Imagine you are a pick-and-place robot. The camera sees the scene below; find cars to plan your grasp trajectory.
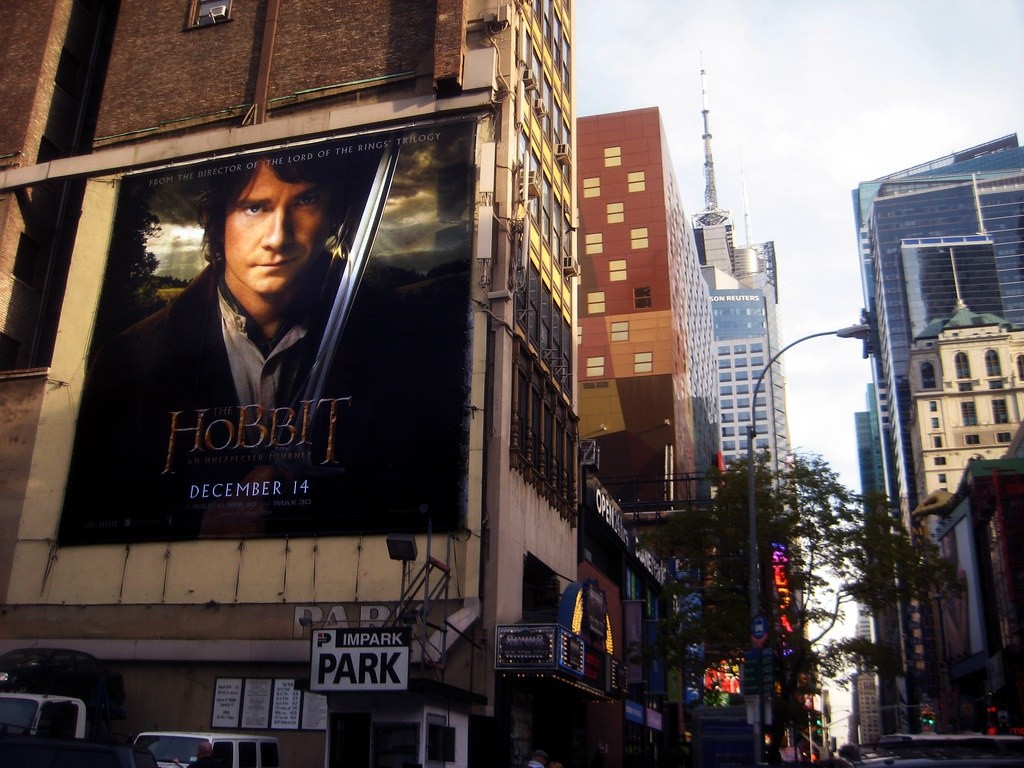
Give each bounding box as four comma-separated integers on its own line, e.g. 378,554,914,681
0,648,126,705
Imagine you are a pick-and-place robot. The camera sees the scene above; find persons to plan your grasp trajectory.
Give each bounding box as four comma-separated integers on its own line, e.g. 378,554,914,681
941,528,970,661
912,489,956,522
187,740,227,768
527,748,562,768
106,144,455,531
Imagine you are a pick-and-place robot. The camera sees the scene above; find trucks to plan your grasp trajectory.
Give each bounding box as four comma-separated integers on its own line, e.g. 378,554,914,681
0,692,87,740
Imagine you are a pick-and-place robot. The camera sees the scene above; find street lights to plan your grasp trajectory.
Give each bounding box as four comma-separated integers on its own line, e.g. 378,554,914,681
747,324,871,768
819,687,930,746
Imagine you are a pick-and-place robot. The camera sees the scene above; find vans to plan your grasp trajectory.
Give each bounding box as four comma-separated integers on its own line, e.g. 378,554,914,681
134,731,281,768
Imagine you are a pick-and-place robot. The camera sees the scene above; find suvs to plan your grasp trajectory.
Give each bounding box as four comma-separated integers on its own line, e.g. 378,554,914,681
0,723,157,768
